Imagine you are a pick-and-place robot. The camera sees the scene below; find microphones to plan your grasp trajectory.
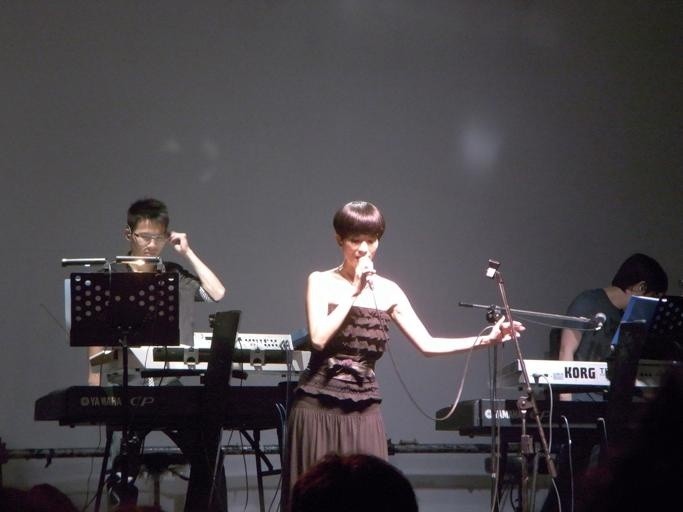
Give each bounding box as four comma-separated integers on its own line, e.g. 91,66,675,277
359,256,375,291
594,312,607,329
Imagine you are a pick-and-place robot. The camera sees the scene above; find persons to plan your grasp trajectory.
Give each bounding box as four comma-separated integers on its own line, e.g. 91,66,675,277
88,198,226,511
280,202,527,512
289,451,419,512
540,253,669,512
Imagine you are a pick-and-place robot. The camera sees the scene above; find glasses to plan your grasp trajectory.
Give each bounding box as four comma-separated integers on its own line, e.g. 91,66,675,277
133,231,166,241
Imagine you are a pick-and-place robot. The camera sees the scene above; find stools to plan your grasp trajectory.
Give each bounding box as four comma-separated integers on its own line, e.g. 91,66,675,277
114,452,190,511
486,453,557,511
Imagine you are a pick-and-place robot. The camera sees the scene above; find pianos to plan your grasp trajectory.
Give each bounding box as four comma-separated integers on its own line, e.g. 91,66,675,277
34,382,299,432
487,359,683,394
436,399,683,443
88,343,312,383
